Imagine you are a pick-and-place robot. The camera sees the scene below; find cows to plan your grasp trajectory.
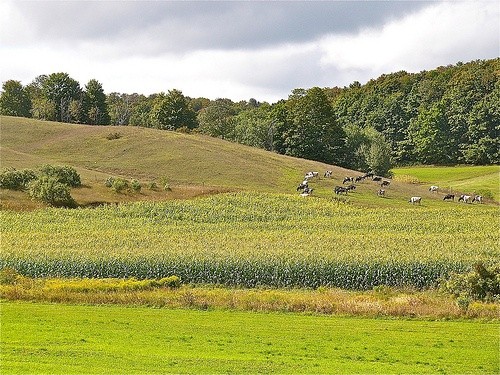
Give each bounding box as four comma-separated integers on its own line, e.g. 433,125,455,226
381,180,392,189
428,185,440,195
376,189,385,198
324,170,333,178
373,177,382,184
301,188,316,196
304,171,321,182
296,181,309,192
443,194,456,203
342,172,375,184
333,185,357,196
409,197,422,206
472,195,483,204
458,194,472,204
